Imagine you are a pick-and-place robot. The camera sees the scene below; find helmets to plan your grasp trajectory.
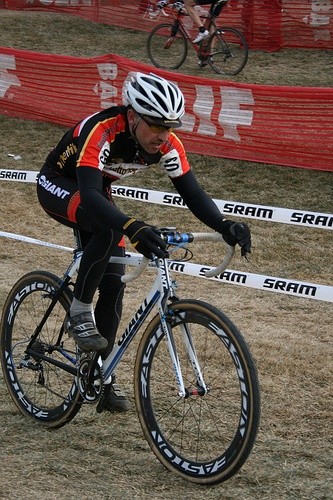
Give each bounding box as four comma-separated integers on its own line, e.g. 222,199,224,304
122,71,186,129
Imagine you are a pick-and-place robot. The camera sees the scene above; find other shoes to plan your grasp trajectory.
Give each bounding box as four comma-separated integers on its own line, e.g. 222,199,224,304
197,57,214,66
192,30,210,43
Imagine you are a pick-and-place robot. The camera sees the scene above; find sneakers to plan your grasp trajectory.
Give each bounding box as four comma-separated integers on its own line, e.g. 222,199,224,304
63,309,109,349
103,384,132,412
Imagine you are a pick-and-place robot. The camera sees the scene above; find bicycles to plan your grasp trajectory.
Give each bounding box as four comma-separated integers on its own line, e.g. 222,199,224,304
0,227,259,486
147,2,249,75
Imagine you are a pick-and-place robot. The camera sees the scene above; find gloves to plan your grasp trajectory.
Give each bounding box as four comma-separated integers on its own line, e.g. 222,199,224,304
216,220,251,257
117,217,167,259
156,1,166,10
173,2,182,10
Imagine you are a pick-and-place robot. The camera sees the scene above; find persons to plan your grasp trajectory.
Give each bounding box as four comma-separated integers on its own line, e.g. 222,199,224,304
37,73,251,409
157,0,226,65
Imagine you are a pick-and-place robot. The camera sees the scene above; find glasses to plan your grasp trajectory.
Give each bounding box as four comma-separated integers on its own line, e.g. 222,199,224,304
137,115,174,133
